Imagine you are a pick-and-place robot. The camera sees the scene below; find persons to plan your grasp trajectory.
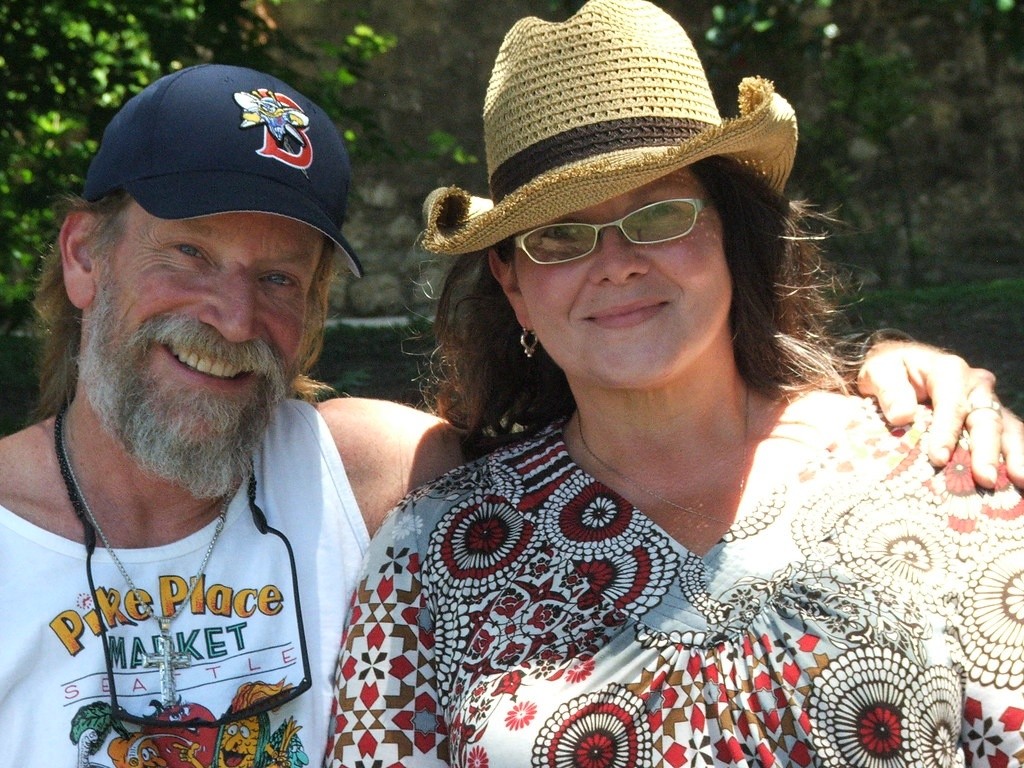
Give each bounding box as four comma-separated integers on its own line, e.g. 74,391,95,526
1,66,1023,768
323,0,1024,768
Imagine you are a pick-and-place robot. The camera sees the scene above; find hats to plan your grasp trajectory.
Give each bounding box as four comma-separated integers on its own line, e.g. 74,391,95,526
420,0,799,256
85,64,365,278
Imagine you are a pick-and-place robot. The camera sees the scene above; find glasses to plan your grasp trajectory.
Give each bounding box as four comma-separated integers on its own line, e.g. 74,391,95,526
78,502,312,728
514,196,717,265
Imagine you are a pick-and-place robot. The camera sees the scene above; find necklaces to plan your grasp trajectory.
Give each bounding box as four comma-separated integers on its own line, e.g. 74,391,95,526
577,385,749,525
60,420,235,709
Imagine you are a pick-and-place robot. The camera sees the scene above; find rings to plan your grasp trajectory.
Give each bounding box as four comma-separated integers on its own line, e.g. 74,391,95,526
967,399,1003,418
